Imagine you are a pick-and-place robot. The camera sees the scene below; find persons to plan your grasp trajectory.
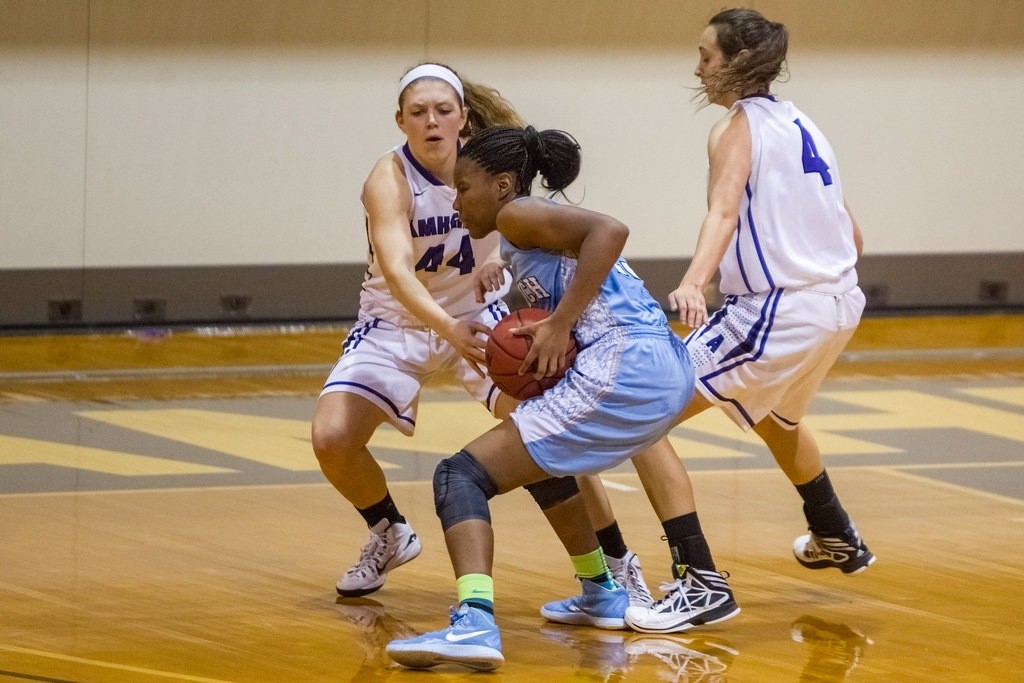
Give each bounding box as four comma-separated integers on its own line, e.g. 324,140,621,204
385,124,695,674
624,8,878,633
310,63,653,605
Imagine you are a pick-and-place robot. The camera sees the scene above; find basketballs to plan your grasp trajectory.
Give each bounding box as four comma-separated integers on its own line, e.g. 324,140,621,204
485,307,580,399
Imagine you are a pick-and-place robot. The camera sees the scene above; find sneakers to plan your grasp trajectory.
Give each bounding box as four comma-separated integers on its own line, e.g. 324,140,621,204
385,602,505,671
788,615,873,666
624,562,741,634
335,516,422,597
793,523,877,577
541,630,627,667
335,594,423,668
540,578,631,629
623,636,740,683
603,549,655,610
